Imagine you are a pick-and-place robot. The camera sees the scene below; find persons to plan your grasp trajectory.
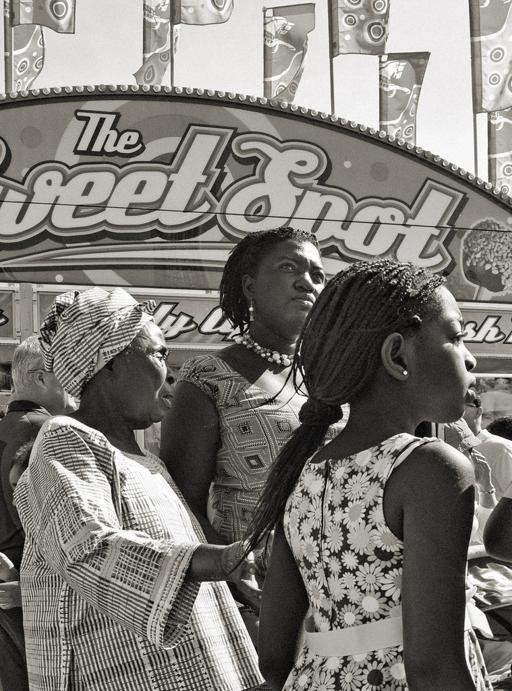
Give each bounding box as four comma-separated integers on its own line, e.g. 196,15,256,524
1,228,510,690
